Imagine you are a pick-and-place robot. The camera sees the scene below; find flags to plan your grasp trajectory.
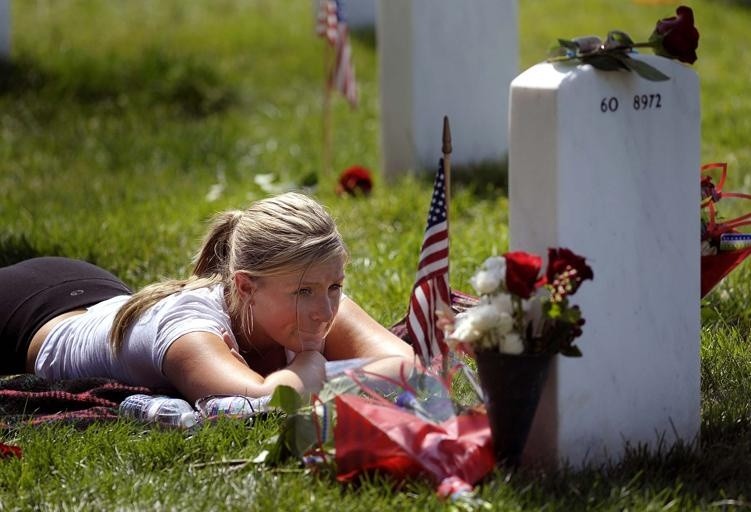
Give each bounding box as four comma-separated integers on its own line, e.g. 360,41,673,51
406,158,454,369
316,0,360,112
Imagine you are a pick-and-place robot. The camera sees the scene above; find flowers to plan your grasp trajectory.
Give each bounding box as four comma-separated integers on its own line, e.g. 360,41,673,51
335,165,373,199
443,245,594,481
254,345,495,511
701,161,751,302
542,7,700,82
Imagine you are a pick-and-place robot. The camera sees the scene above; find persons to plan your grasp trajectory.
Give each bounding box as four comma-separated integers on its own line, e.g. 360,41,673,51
0,190,423,412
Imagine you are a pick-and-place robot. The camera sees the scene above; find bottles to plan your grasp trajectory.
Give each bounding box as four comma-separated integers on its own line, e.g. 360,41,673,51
204,395,278,413
119,394,196,428
